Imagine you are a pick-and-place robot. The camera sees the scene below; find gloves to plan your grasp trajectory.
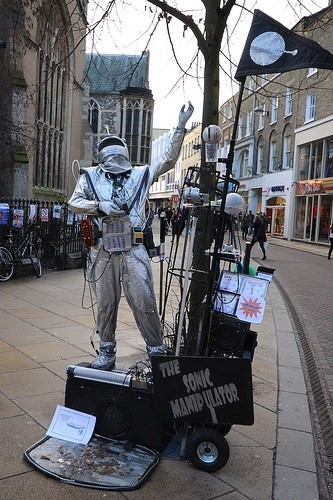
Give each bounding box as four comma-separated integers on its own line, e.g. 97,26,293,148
99,201,125,217
178,100,195,129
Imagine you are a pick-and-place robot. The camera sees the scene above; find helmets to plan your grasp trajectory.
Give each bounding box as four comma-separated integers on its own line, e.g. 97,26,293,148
97,136,132,173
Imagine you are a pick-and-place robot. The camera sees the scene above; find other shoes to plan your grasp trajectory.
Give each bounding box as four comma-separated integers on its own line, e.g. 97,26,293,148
92,340,117,370
262,256,267,260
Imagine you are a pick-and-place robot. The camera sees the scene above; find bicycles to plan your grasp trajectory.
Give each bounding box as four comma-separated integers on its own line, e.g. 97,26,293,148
0,226,42,282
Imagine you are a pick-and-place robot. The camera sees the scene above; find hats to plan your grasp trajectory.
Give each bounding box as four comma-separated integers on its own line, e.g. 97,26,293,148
255,215,261,221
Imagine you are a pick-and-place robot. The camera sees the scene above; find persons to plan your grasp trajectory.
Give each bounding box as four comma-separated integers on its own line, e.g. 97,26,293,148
148,206,269,243
328,221,333,260
67,101,194,371
251,215,267,260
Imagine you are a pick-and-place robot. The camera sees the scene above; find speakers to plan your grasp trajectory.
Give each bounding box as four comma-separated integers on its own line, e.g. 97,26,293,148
64,376,171,455
208,312,251,358
